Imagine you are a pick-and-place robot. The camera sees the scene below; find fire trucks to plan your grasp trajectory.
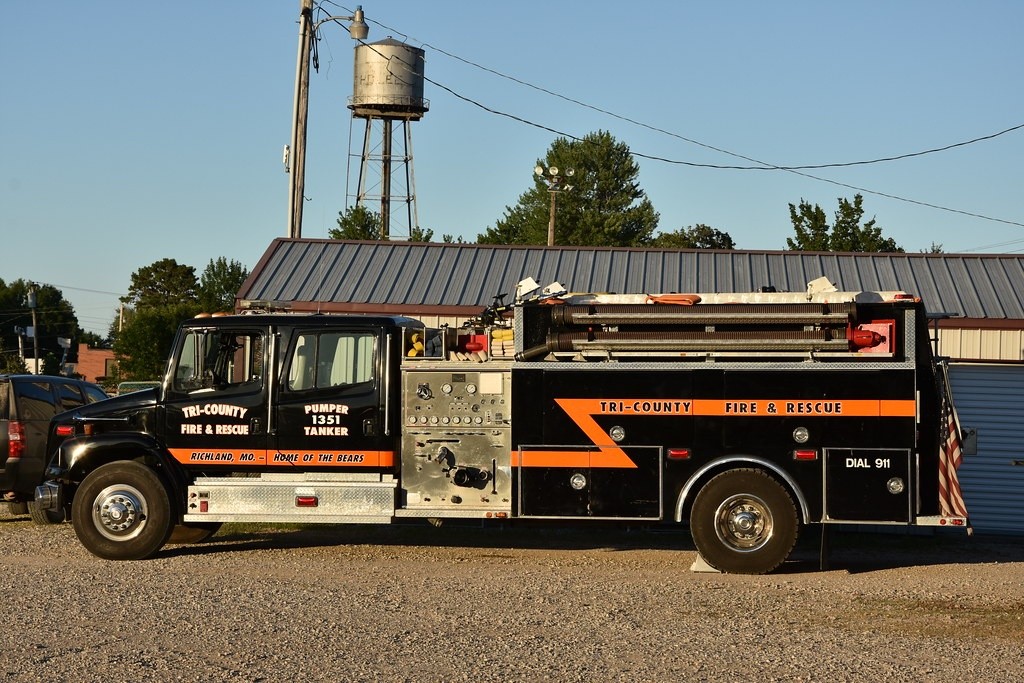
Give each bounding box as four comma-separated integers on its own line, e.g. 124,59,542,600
34,277,978,578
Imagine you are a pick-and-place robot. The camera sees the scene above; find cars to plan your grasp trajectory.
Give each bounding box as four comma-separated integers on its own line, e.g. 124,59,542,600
0,374,110,525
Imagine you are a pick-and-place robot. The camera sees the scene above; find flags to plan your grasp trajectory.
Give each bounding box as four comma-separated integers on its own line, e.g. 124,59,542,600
939,381,968,519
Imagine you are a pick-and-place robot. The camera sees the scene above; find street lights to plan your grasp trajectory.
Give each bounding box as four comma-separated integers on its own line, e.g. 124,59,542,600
290,4,369,239
533,165,576,247
